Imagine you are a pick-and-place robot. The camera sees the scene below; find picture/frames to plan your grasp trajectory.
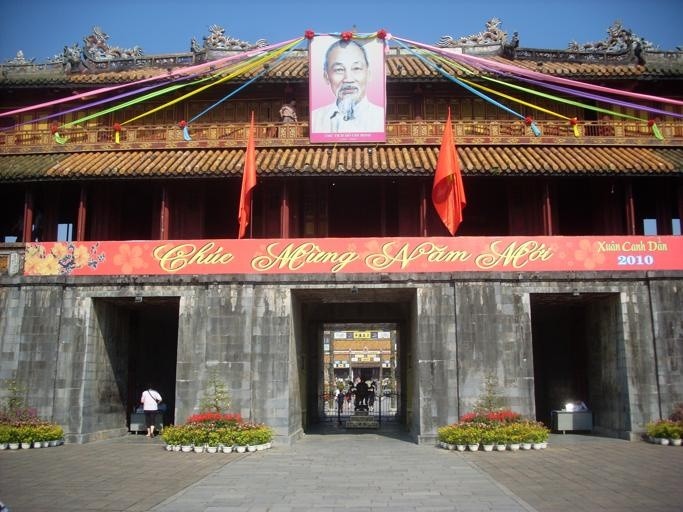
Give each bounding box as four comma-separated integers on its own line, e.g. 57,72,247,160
309,33,388,143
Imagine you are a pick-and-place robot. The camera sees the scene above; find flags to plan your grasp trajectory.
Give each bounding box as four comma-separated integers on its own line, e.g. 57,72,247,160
431,106,468,236
236,111,256,239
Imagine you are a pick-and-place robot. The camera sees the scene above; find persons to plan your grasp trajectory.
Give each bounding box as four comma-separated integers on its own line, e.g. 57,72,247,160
354,375,368,405
279,98,297,126
333,386,339,410
311,39,383,133
336,388,344,413
140,381,162,438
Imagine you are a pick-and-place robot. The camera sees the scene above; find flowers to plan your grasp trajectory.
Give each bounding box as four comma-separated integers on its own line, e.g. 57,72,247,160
439,372,551,445
159,366,271,447
0,379,64,443
641,402,683,439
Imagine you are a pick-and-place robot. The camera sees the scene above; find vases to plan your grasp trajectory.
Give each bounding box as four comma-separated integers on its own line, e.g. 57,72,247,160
647,435,683,446
167,442,273,453
441,443,548,450
0,441,64,450
